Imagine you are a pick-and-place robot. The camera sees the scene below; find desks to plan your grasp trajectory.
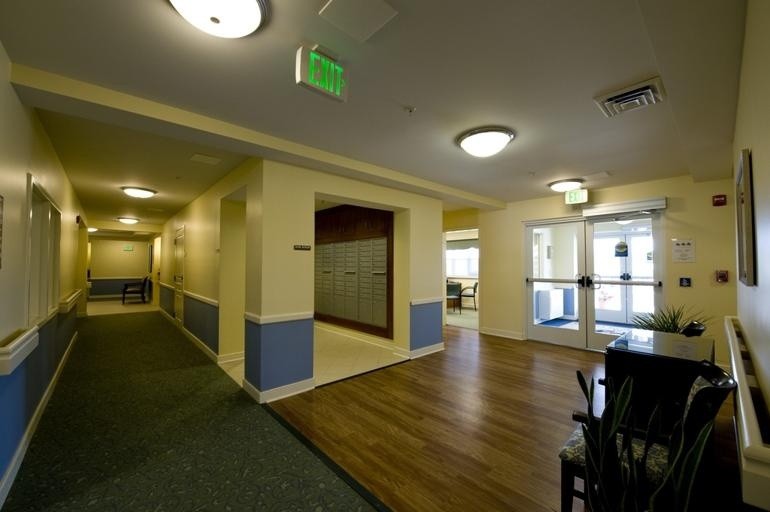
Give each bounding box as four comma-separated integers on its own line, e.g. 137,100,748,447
605,328,717,446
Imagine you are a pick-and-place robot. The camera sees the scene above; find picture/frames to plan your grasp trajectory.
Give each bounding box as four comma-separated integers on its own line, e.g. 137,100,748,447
734,146,755,289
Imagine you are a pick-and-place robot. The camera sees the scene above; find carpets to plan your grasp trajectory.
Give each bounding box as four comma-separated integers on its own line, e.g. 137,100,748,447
5,309,396,512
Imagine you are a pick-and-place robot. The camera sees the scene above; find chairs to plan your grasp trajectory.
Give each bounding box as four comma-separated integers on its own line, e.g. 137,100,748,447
121,274,150,306
556,357,738,512
444,282,479,315
679,318,707,337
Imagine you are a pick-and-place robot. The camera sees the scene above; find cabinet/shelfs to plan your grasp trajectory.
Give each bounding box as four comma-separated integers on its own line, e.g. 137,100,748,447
314,236,387,328
